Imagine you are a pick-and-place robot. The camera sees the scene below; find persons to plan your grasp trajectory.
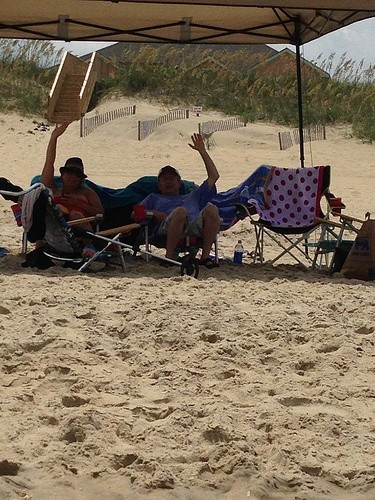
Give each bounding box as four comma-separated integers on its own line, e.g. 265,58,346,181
40,122,104,258
132,133,220,269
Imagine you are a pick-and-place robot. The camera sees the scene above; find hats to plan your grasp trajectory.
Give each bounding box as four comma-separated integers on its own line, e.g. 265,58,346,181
59,157,84,175
157,165,181,178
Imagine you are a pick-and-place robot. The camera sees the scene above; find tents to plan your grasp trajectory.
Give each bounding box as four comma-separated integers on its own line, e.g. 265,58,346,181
0,0,375,258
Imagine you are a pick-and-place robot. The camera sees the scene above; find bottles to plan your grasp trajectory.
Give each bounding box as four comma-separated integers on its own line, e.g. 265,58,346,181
11,204,22,226
97,251,107,263
233,240,244,263
365,212,370,221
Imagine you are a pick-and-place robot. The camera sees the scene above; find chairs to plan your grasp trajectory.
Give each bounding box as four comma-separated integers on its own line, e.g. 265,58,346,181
0,166,375,282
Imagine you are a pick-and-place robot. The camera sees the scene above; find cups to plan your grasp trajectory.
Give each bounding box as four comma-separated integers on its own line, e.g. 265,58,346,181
133,204,145,224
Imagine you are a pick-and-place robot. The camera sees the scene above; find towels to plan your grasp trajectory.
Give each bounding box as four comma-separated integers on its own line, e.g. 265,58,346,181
251,165,324,225
21,185,49,243
31,175,186,237
192,167,269,228
